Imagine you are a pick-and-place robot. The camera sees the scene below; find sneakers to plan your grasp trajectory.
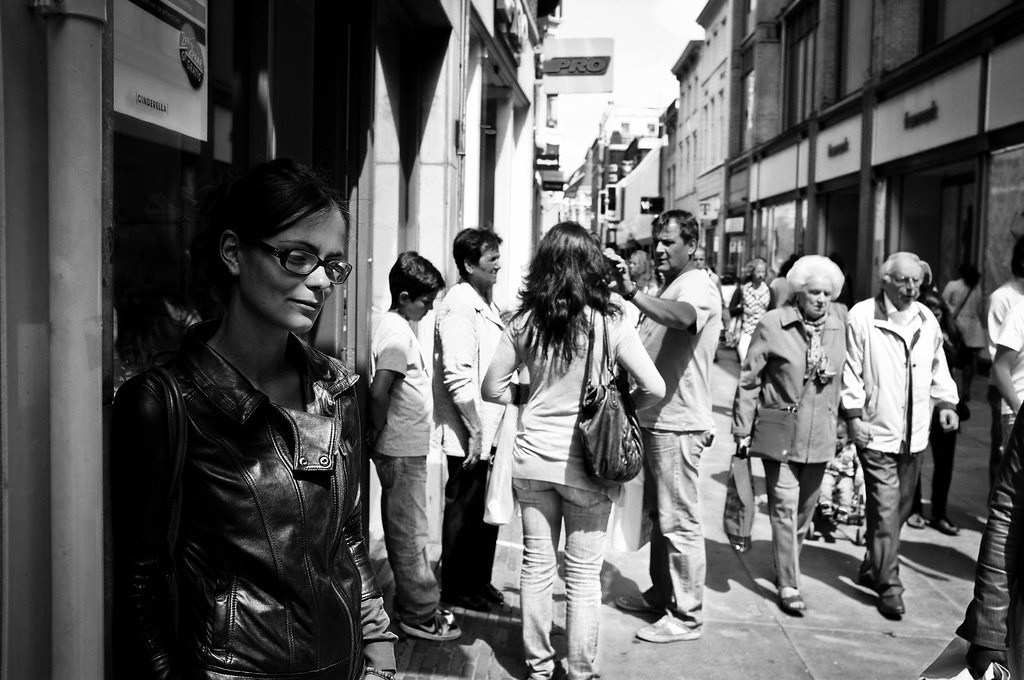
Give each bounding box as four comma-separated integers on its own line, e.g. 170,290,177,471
436,609,455,624
398,612,461,641
615,595,653,612
638,616,704,643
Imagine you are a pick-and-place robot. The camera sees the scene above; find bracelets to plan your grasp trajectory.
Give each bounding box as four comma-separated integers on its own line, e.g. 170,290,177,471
623,287,638,301
364,667,396,680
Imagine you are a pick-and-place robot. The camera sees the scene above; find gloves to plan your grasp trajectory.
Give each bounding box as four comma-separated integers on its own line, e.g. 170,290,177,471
965,643,1007,679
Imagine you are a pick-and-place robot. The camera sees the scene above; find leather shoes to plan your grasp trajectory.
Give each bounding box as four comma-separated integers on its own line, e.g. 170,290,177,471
858,577,884,595
879,595,905,613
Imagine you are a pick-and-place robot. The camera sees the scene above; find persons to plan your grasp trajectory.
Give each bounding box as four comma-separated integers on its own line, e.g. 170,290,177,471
840,252,960,614
954,401,1024,680
919,260,985,404
435,228,504,612
479,223,666,680
694,246,733,348
629,250,658,297
113,160,399,680
370,252,463,641
603,209,722,642
729,258,798,366
731,255,848,611
984,234,1024,482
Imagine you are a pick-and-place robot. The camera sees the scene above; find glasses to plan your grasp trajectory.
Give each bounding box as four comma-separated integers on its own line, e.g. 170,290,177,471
262,243,352,284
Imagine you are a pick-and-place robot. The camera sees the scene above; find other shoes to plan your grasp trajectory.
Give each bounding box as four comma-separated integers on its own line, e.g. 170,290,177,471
821,506,832,516
442,594,492,610
932,516,960,535
907,511,925,528
837,510,849,523
483,585,507,603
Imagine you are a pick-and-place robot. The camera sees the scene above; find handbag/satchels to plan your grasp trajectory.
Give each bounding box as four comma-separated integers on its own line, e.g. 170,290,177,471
104,366,198,680
750,407,798,463
577,306,644,481
619,467,652,550
728,287,744,316
723,446,754,552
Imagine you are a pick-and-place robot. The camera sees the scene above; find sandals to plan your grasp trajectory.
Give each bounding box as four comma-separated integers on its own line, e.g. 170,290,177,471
778,586,808,611
